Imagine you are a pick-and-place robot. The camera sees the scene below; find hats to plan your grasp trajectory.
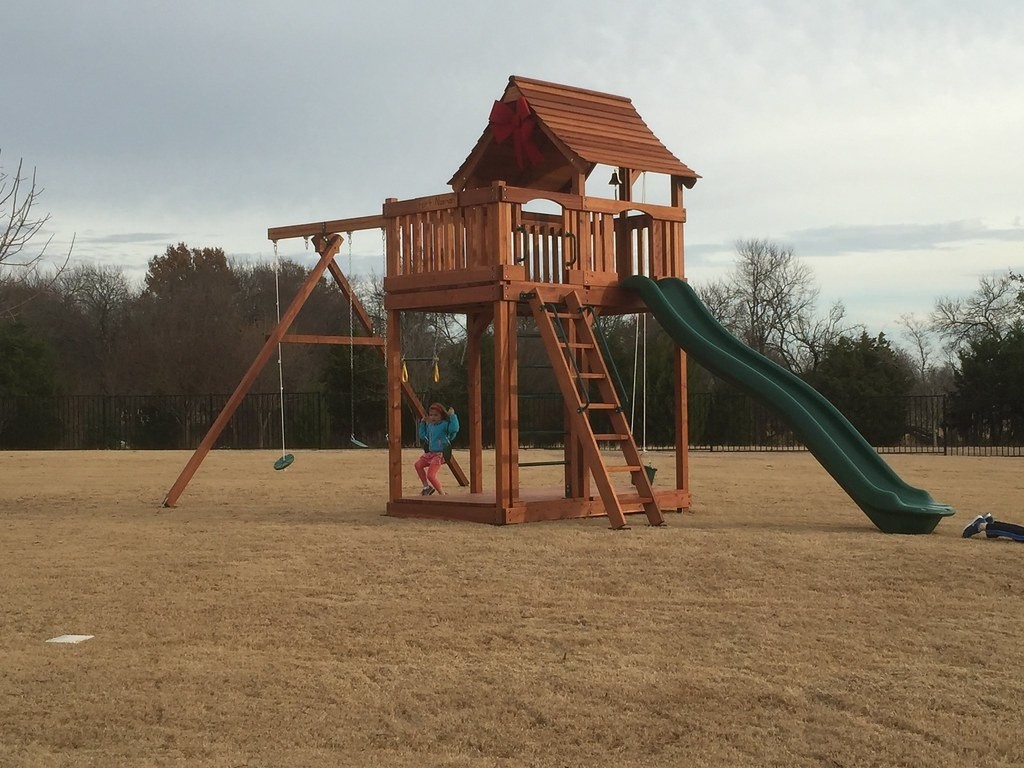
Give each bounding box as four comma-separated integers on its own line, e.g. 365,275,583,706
431,402,448,416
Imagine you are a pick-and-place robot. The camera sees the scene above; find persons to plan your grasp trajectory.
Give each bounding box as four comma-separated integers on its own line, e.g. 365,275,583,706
414,402,460,495
962,512,1024,543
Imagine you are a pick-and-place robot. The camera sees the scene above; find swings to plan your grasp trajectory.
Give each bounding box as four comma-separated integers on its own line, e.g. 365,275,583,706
347,226,389,448
424,312,470,465
273,235,310,470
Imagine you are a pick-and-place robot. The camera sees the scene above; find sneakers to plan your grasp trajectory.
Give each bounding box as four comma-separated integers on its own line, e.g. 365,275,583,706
962,515,986,538
421,485,435,495
984,513,995,523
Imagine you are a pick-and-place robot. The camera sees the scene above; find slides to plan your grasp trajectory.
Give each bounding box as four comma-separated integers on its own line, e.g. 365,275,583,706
626,271,960,537
